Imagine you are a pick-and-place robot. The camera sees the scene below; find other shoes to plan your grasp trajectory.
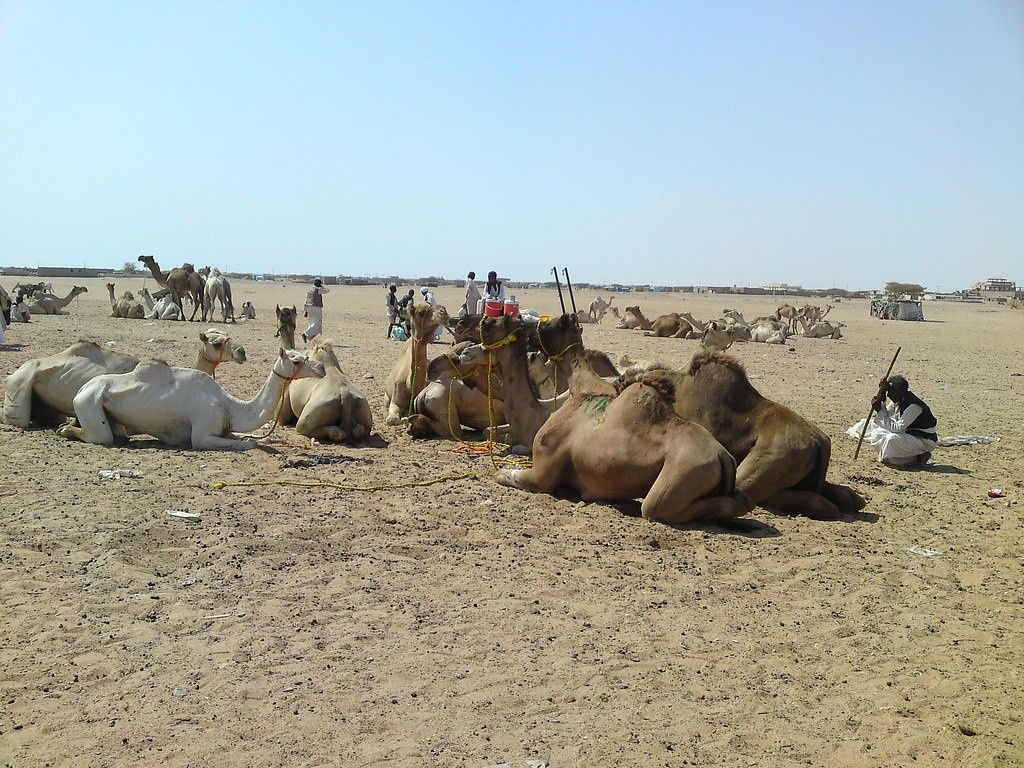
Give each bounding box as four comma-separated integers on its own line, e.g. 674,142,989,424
908,452,932,472
302,333,307,343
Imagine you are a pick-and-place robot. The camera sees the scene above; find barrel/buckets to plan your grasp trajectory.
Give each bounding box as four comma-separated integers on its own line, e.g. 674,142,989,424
485,299,502,318
504,300,519,320
540,315,549,322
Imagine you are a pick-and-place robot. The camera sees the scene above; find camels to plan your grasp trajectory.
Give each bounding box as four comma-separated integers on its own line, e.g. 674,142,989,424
578,297,845,354
272,304,374,443
56,348,328,451
383,302,867,521
0,328,247,429
106,255,256,324
12,279,89,315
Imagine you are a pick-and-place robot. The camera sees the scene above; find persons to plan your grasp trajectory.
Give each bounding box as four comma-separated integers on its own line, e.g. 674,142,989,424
386,285,414,339
872,375,937,465
420,287,442,339
10,298,31,322
240,301,256,319
302,279,329,342
483,271,505,307
220,301,235,318
382,282,387,288
458,272,481,316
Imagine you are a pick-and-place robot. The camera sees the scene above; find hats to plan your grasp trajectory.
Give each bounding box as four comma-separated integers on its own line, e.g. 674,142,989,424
887,375,909,395
420,286,428,293
